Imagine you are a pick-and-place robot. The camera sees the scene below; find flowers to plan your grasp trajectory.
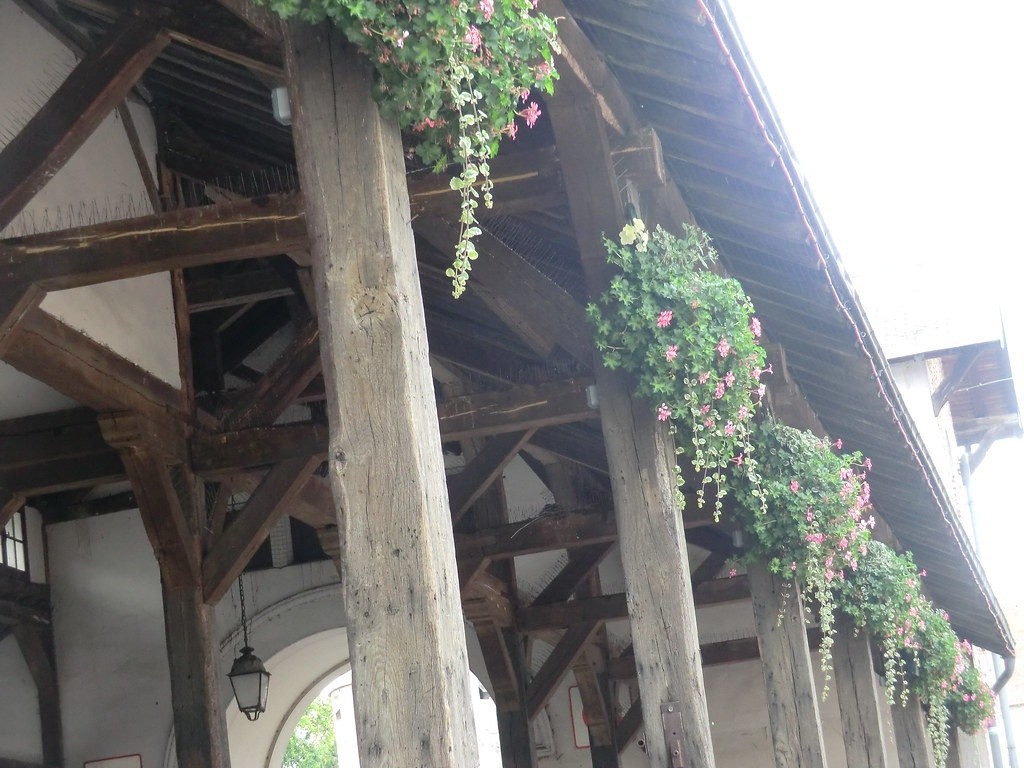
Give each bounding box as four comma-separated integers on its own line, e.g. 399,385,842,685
250,0,561,300
587,219,997,768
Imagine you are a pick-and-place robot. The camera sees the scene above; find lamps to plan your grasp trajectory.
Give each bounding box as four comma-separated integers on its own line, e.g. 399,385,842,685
226,477,271,721
